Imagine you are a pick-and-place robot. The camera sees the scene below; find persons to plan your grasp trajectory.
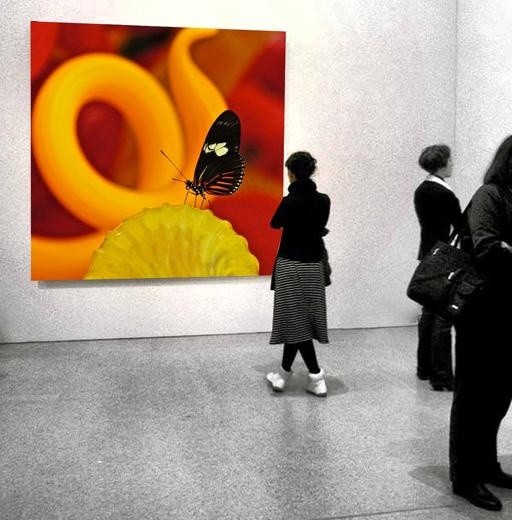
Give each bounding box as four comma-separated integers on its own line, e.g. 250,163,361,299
267,152,331,397
451,136,512,512
417,146,461,392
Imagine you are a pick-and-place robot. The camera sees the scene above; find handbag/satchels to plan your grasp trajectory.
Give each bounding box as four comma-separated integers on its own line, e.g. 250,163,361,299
405,239,493,324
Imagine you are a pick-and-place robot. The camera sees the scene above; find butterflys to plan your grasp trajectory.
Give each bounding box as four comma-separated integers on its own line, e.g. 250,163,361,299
160,109,248,210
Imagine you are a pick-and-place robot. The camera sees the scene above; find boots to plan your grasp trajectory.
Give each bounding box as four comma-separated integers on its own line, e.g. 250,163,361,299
305,368,327,398
267,366,294,393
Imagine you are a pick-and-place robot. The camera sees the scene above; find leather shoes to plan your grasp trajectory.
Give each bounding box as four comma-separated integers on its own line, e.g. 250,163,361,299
416,370,456,394
447,477,503,513
474,463,512,492
